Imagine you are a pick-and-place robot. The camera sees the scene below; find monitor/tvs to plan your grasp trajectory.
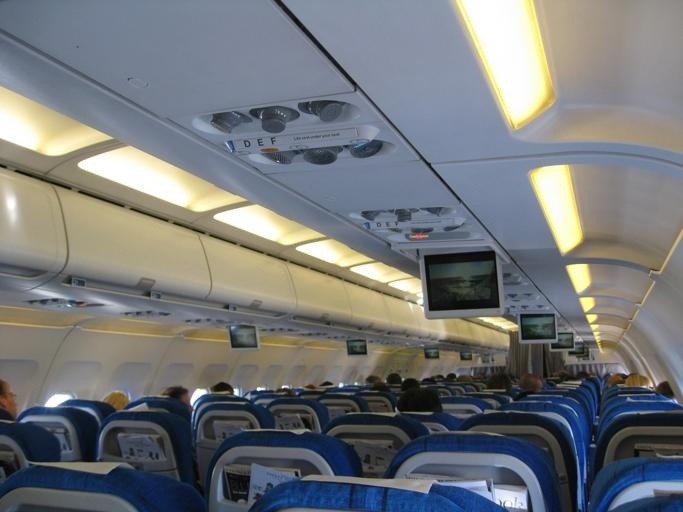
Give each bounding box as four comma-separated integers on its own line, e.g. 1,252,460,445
423,348,439,359
517,311,558,343
419,248,504,318
460,352,472,361
549,331,590,361
346,338,368,357
227,323,260,351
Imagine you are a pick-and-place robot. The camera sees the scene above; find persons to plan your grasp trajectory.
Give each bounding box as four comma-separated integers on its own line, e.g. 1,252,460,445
0,378,19,423
488,370,589,395
159,384,194,414
607,373,674,398
304,373,456,413
100,389,129,414
207,380,235,395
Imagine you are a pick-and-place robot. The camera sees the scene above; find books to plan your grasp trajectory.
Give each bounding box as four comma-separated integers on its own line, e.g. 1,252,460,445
273,414,308,431
224,437,532,512
48,424,69,451
0,449,20,481
631,443,683,456
115,431,168,460
211,418,252,441
